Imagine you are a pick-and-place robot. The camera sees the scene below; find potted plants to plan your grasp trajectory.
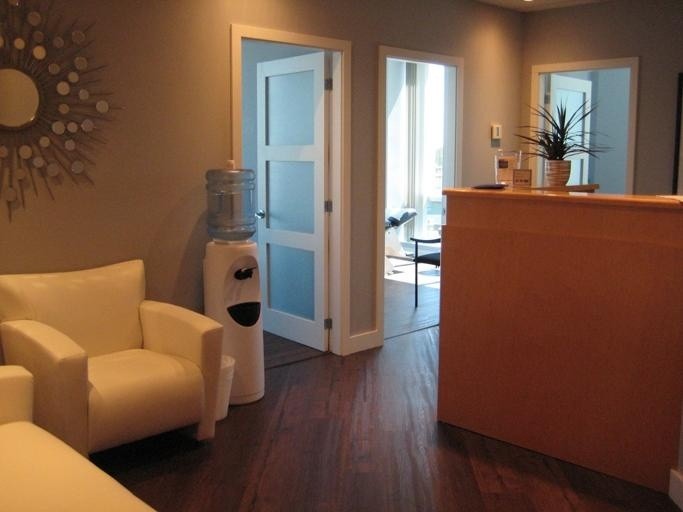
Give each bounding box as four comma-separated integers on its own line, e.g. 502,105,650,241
512,94,613,187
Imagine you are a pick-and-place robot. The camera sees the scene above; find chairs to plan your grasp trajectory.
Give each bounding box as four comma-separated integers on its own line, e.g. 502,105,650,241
1,257,224,461
1,361,155,511
408,234,442,307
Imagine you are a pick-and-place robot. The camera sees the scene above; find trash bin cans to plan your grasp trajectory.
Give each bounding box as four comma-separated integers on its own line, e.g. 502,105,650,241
216,355,235,422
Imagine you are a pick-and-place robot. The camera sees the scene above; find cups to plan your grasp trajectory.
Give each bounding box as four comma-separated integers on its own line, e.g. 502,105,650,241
494,149,521,187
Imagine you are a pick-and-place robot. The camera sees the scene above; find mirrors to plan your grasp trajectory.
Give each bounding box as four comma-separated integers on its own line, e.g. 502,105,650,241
0,63,45,133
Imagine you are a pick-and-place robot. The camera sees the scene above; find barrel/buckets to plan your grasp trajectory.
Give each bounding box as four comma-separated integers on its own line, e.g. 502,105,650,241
205,168,256,241
